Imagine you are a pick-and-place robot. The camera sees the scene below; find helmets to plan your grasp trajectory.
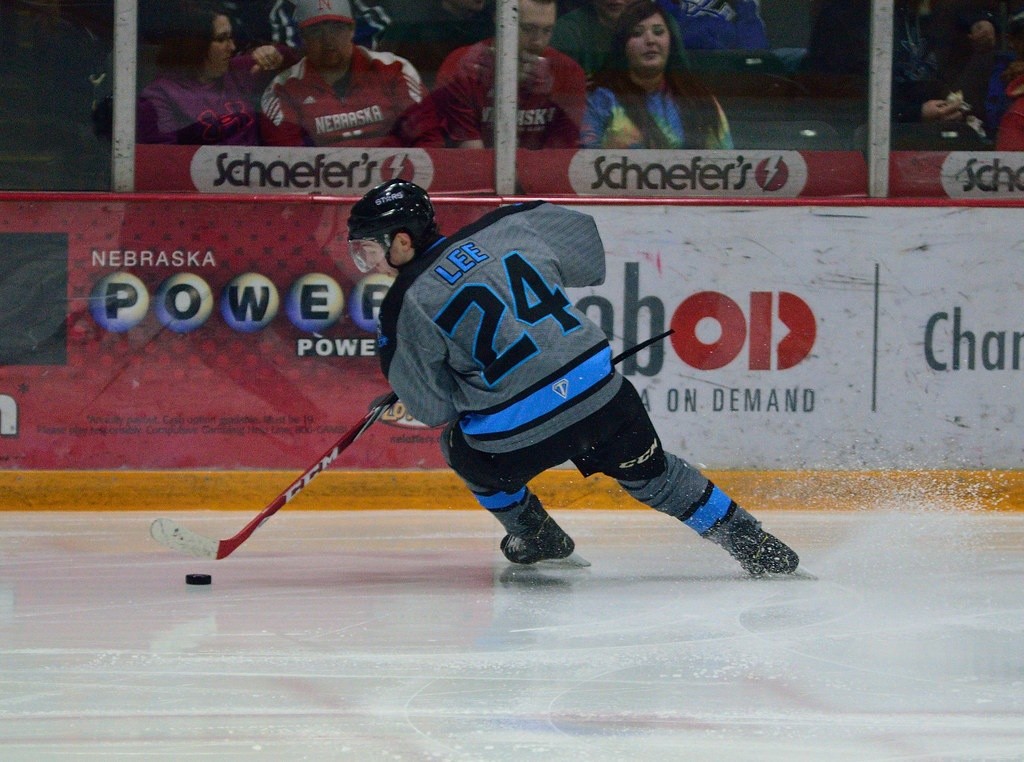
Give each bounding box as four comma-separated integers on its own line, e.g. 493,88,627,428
348,179,438,248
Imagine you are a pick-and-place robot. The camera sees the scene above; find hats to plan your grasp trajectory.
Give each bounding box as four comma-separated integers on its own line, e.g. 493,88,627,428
291,0,355,29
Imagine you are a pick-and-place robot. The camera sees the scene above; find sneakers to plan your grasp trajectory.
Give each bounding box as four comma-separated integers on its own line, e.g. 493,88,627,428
740,535,799,574
498,516,575,565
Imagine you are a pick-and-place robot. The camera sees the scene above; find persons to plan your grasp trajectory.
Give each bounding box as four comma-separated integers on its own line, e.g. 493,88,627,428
136,1,300,146
576,0,735,150
60,0,1024,149
257,0,445,149
433,0,588,149
345,176,815,583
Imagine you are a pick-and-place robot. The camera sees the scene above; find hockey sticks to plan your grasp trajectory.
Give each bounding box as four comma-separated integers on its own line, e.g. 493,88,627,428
148,390,400,562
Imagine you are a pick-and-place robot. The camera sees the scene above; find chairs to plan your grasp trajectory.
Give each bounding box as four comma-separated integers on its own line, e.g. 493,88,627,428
689,47,783,76
851,122,979,151
729,118,842,153
0,12,95,157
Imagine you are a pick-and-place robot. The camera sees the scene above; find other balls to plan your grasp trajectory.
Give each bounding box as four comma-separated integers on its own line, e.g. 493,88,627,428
185,573,212,586
